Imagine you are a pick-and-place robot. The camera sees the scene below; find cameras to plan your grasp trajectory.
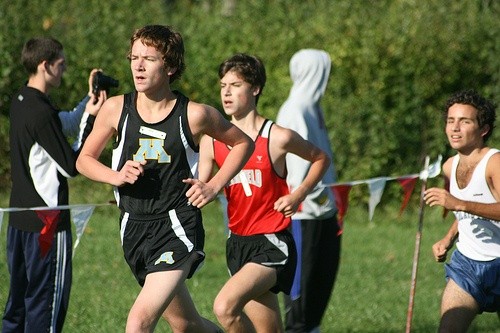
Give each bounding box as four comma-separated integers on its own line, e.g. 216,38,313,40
92,72,119,105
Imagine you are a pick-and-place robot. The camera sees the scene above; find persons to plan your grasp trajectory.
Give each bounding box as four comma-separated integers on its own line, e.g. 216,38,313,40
197,53,330,333
277,49,342,333
3,36,108,333
75,26,254,333
421,90,500,333
